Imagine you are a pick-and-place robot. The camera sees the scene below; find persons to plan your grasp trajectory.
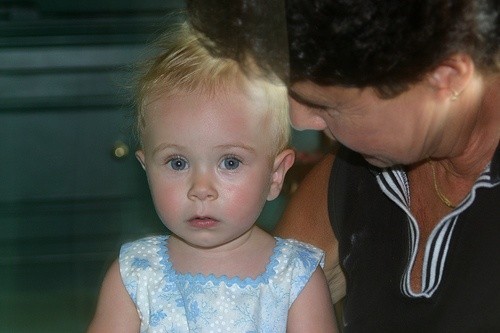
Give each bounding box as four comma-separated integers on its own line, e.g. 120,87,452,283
86,20,340,333
180,0,500,333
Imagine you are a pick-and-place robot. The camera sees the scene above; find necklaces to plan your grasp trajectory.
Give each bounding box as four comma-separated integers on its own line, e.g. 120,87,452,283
426,155,469,209
